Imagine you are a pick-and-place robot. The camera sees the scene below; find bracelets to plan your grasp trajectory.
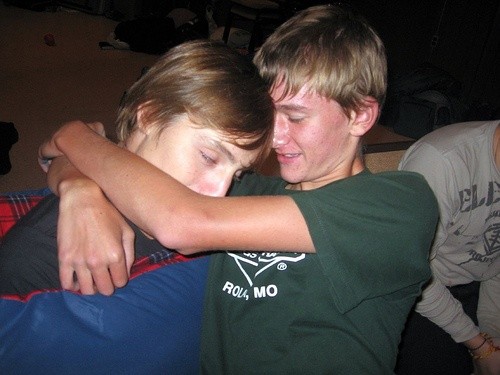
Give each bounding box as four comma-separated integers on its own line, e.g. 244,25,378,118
467,333,495,361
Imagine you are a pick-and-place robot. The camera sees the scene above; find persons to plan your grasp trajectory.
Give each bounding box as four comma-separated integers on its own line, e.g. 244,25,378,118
395,118,500,375
37,4,438,375
0,36,275,298
